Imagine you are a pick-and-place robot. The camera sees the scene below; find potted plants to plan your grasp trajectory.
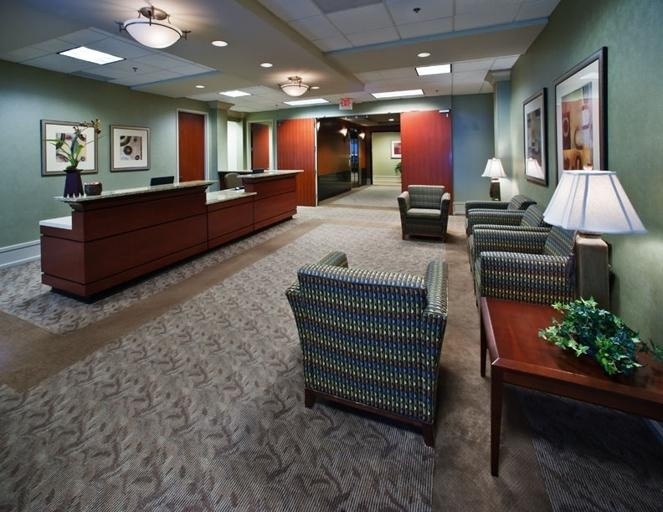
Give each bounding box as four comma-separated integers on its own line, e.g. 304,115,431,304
44,117,103,171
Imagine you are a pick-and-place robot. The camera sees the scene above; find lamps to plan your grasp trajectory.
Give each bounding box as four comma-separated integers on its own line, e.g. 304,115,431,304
541,163,649,314
478,156,506,201
112,7,189,49
276,75,311,98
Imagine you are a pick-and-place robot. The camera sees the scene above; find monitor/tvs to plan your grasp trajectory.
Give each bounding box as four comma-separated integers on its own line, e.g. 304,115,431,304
253,169,264,174
151,176,174,186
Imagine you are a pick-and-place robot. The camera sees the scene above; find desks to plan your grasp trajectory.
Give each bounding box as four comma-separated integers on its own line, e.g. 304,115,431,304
474,292,662,479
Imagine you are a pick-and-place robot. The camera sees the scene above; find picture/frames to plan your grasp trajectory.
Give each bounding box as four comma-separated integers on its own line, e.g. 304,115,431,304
551,45,610,191
37,118,99,180
104,122,151,173
389,138,402,160
520,85,550,191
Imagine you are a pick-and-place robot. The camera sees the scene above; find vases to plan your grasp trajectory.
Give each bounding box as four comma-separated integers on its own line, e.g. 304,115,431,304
62,168,85,198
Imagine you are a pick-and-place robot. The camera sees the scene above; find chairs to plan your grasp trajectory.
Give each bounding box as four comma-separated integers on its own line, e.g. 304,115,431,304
284,250,450,453
467,223,579,306
463,191,538,238
394,184,453,243
224,171,243,189
466,203,548,271
